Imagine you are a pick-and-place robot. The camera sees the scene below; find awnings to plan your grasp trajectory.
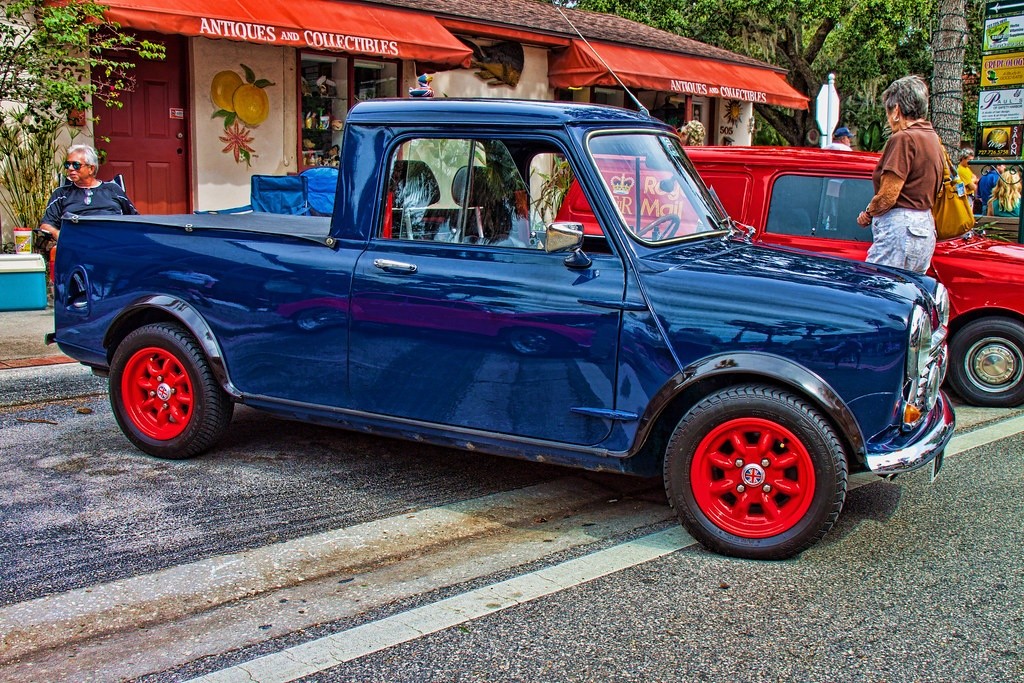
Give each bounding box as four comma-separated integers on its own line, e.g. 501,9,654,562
35,0,473,69
548,39,810,112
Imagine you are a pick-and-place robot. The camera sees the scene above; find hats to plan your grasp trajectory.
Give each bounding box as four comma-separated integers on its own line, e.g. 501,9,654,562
835,127,854,138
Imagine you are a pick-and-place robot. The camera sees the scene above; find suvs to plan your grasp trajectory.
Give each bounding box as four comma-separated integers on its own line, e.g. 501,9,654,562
549,144,1024,409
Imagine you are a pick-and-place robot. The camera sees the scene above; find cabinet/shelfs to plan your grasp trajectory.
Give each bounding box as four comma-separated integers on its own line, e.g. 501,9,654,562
296,47,404,173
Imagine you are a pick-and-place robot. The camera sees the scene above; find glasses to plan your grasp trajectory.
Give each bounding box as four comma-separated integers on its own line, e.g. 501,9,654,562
63,161,90,170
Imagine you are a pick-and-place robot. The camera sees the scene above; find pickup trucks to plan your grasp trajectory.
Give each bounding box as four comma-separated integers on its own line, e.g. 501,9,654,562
44,7,958,562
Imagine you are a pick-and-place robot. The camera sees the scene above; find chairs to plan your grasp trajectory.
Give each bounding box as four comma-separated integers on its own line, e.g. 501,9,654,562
194,175,311,216
33,173,125,260
389,160,439,240
451,166,503,237
782,208,813,235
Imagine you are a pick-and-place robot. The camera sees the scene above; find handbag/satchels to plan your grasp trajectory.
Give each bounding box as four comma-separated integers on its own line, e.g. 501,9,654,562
932,137,975,239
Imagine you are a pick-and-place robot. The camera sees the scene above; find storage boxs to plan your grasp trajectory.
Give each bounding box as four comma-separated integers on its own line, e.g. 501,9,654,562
0,253,47,311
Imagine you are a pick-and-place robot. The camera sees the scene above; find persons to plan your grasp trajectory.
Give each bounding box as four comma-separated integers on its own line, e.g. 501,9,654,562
956,148,980,215
856,75,946,275
977,156,1007,216
987,171,1022,217
821,128,856,151
40,144,141,244
